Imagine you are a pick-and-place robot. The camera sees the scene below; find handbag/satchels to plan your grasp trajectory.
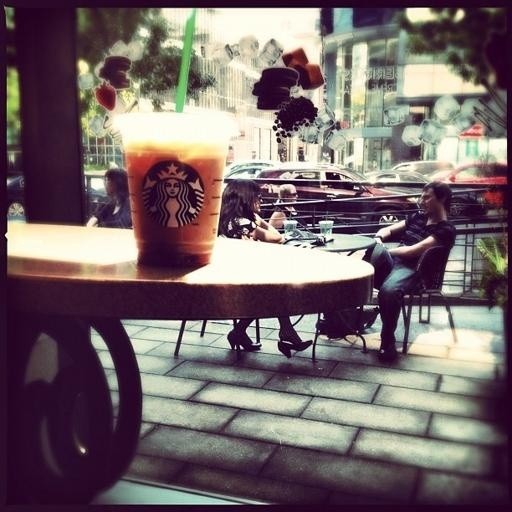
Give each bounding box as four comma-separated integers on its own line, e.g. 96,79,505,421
322,307,380,339
376,340,396,362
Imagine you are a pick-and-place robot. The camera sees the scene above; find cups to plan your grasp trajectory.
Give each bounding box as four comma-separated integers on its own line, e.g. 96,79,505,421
282,220,298,236
319,220,334,238
113,108,237,266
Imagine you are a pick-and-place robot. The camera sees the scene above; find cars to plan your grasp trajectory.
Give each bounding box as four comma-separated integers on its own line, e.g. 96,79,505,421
224,160,507,232
8,175,110,218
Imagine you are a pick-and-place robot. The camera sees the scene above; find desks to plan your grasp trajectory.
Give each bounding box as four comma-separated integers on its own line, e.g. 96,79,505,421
279,228,375,363
0,223,374,511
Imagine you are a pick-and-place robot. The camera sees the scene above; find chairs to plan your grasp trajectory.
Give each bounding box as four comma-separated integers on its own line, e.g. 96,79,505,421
374,234,454,355
175,218,260,357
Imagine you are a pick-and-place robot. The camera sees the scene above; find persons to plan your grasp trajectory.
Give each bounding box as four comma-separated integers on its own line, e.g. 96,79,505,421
6,156,25,215
217,179,313,358
363,181,456,362
268,183,308,234
86,168,132,227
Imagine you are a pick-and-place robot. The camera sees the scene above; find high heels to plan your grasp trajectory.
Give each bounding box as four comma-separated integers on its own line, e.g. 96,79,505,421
277,340,312,358
227,330,262,352
